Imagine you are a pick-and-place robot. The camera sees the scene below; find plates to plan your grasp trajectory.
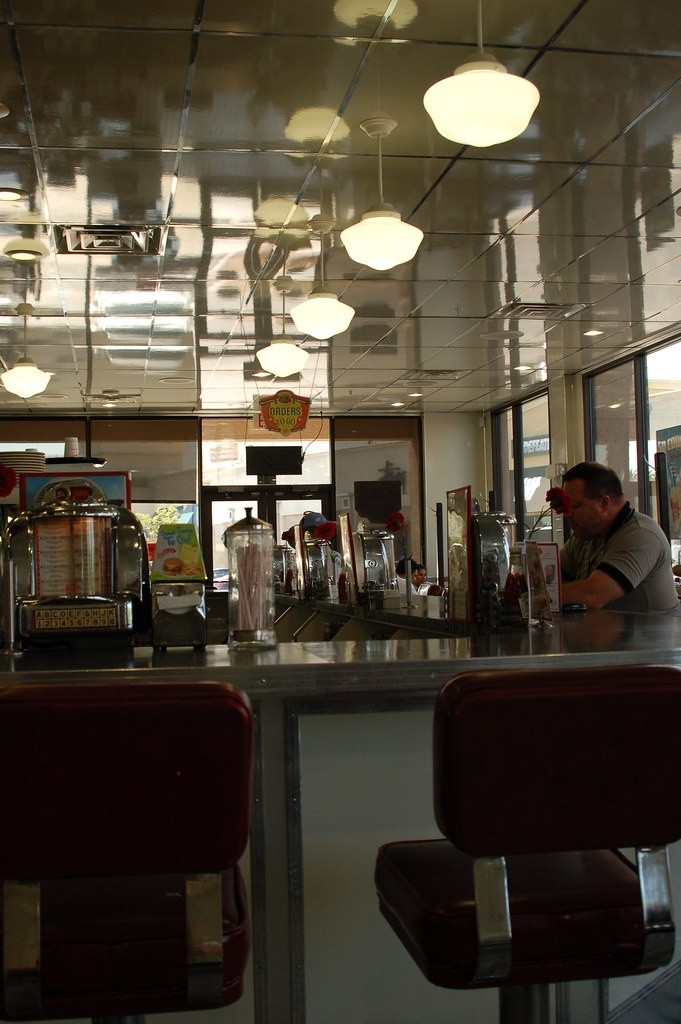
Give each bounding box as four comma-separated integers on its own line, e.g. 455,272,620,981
0,451,47,483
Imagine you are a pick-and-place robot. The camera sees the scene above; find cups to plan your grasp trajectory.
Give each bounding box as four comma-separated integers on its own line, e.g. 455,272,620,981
64,437,80,457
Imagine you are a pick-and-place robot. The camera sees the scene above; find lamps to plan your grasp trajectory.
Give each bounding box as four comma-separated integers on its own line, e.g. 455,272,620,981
422,0,540,149
340,119,424,270
290,221,356,340
256,282,310,377
0,307,51,399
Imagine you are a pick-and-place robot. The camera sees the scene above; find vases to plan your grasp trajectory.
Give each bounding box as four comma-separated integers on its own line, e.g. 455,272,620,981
333,561,336,581
401,559,419,609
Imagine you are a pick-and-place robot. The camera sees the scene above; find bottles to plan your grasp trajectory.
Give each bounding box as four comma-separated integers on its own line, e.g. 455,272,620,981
338,566,349,603
225,508,278,650
285,561,296,596
503,547,529,629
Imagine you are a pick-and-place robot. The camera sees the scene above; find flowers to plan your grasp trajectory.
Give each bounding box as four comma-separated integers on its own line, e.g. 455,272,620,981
385,511,413,558
281,531,292,551
523,487,573,540
314,521,338,562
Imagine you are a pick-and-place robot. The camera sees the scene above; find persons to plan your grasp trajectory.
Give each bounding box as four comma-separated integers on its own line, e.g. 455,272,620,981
389,558,418,595
413,564,431,596
428,585,447,597
309,548,353,582
559,462,681,616
550,610,676,653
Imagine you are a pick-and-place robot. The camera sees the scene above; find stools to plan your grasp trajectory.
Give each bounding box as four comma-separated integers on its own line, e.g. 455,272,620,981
375,664,681,1024
0,680,255,1024
